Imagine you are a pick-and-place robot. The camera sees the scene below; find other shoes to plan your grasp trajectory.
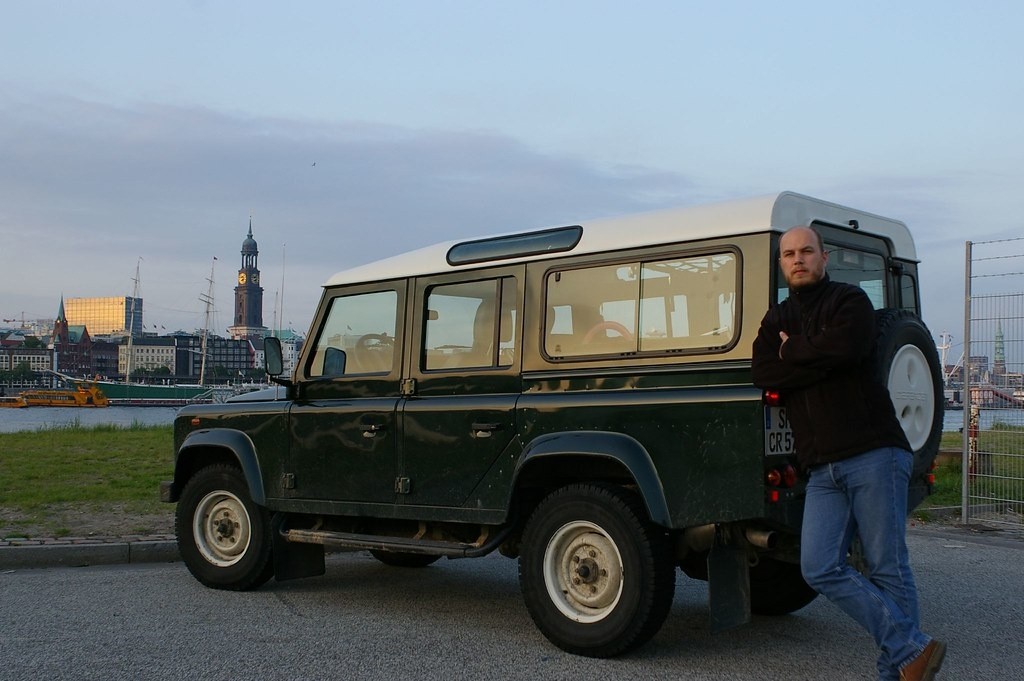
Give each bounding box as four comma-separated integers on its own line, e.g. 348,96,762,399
899,639,947,681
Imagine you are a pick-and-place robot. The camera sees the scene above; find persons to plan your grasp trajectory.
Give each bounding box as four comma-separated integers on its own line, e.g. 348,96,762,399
749,227,948,681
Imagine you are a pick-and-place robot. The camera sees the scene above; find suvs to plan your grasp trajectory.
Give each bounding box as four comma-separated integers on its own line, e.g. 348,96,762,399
157,189,945,659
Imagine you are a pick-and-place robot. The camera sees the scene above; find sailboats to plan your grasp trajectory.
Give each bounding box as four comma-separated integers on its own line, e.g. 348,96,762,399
0,251,261,409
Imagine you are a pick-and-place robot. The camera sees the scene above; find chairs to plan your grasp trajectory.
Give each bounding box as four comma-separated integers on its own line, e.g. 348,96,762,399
442,297,607,368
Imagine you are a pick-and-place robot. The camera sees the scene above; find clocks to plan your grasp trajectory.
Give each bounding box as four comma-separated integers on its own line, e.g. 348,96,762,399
238,273,247,284
251,273,258,284
